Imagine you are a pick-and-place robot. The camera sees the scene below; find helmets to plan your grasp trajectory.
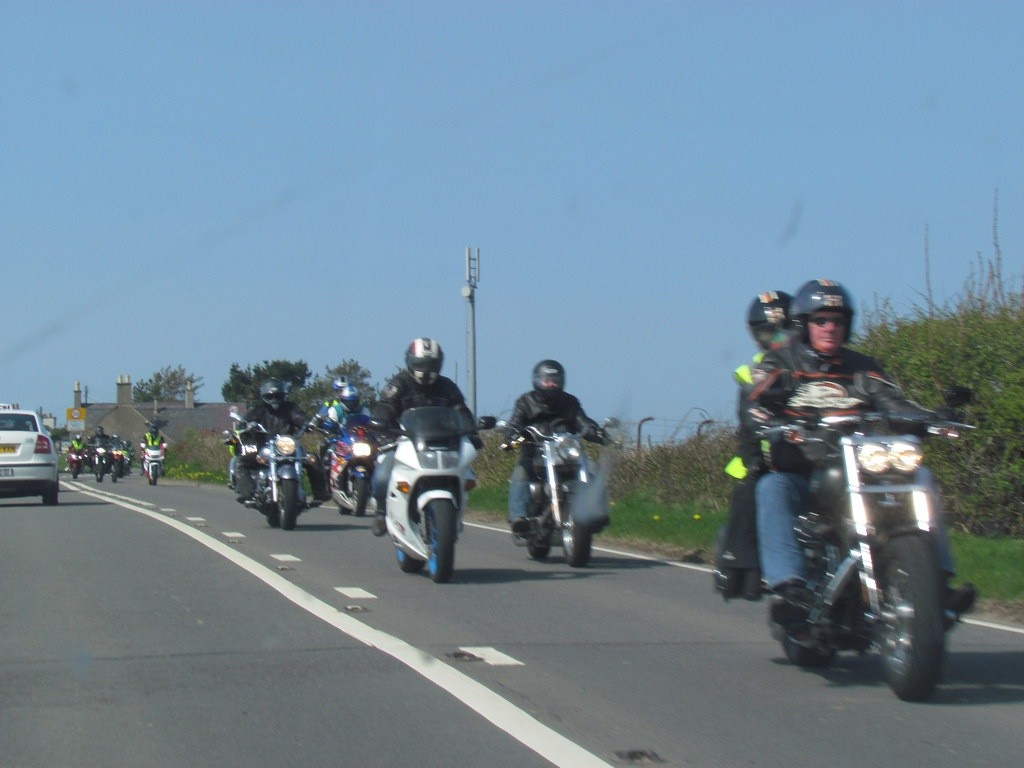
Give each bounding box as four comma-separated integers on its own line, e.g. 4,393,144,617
405,337,443,385
748,290,798,351
112,433,119,439
334,377,350,391
532,360,564,399
341,386,360,407
95,426,103,435
260,377,284,406
76,434,81,441
150,425,158,433
792,279,855,322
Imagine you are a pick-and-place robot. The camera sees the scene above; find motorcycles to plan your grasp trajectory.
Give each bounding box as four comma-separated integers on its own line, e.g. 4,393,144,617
140,442,168,485
489,414,624,568
223,404,328,530
66,437,136,484
748,385,986,704
312,403,380,518
356,401,499,583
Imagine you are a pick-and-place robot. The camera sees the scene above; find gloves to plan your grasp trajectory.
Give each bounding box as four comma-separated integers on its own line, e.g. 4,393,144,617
934,407,957,424
765,419,810,443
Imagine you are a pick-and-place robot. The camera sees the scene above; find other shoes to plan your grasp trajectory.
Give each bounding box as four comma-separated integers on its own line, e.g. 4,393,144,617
741,570,761,600
371,510,387,538
770,596,815,634
314,493,333,501
938,582,977,632
236,497,253,503
512,515,531,539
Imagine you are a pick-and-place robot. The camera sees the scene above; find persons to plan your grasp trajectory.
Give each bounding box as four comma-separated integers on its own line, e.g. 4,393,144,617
711,279,979,632
139,424,167,476
110,434,134,475
228,421,240,487
66,435,89,472
88,427,114,474
369,336,483,537
305,375,375,501
504,360,611,536
233,377,312,504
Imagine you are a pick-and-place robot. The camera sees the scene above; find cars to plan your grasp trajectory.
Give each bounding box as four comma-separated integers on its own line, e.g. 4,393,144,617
0,404,60,507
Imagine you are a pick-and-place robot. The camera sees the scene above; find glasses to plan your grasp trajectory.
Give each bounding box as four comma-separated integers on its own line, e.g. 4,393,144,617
804,314,847,327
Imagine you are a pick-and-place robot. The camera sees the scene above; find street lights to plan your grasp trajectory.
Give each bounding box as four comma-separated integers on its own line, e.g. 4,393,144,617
460,245,482,417
